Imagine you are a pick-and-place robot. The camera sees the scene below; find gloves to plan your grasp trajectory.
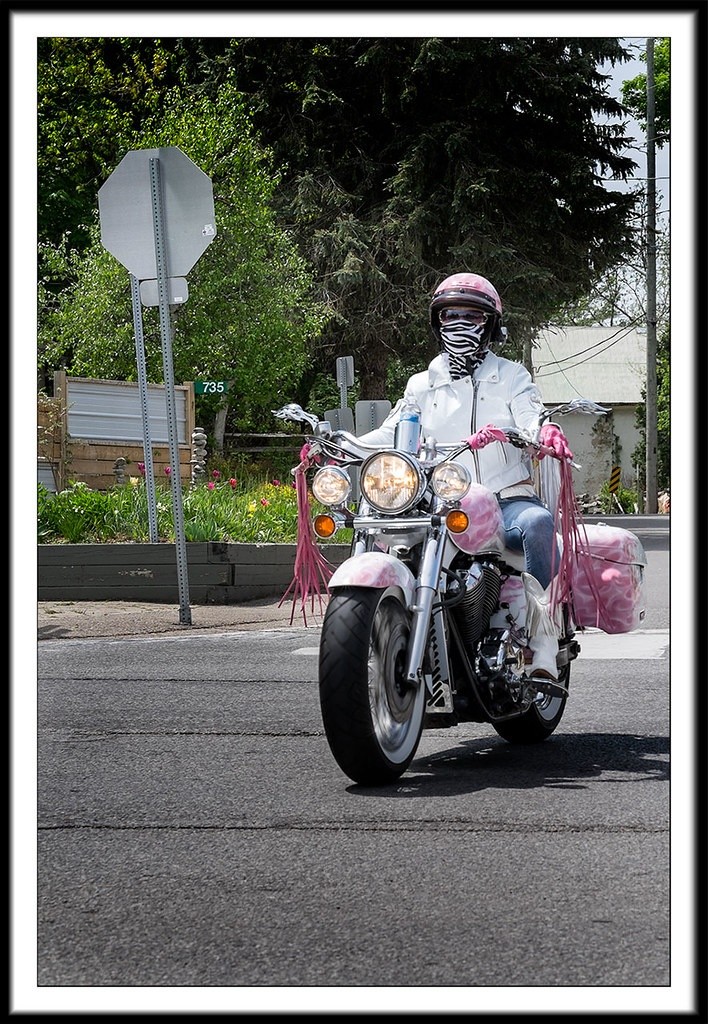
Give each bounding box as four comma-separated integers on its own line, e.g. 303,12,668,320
298,443,334,465
536,426,574,461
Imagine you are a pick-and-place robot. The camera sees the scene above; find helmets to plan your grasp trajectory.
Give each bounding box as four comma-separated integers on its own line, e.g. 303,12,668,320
429,272,504,343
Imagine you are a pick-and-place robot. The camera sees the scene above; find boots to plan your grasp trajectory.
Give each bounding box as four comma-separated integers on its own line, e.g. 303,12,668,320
522,569,561,682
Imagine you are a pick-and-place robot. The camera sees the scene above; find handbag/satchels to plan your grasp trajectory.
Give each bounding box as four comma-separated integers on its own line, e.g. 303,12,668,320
566,522,648,634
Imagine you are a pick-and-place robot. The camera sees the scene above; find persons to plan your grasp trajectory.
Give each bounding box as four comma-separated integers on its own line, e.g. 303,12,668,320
302,272,572,681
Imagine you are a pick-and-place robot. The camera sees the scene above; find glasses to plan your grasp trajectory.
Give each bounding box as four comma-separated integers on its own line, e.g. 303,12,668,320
438,309,492,326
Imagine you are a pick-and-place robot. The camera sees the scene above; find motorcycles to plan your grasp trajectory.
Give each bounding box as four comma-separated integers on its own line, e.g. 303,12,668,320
268,403,585,789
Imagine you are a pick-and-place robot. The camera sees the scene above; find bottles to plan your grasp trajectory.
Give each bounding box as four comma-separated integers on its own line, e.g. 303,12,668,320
397,395,422,454
